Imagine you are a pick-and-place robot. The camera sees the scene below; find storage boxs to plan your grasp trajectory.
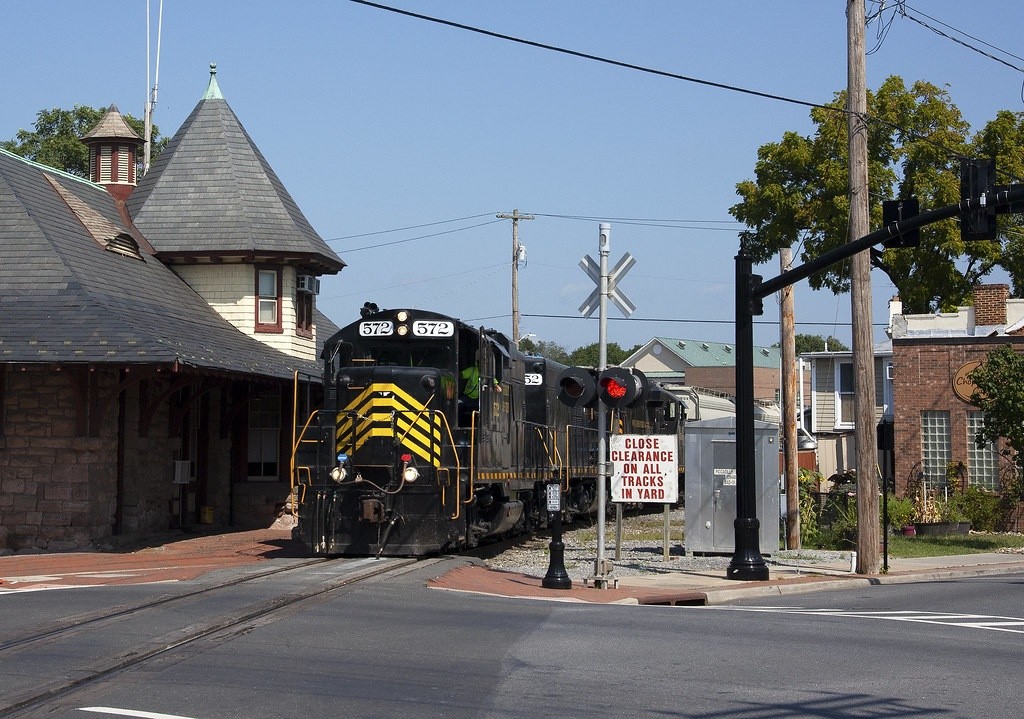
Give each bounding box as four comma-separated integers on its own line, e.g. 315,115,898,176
200,506,214,523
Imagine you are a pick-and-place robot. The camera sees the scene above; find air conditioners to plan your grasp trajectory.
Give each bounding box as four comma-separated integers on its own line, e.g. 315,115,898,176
172,461,190,484
298,274,320,295
886,366,894,379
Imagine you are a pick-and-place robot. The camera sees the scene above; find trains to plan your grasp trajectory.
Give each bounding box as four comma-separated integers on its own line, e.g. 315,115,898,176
289,301,783,559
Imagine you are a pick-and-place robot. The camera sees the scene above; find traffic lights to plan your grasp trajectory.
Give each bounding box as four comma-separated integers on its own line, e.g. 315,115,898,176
596,367,636,410
555,367,597,407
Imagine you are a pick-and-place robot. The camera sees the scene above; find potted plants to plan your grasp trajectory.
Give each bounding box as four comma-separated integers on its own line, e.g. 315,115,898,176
914,494,971,535
